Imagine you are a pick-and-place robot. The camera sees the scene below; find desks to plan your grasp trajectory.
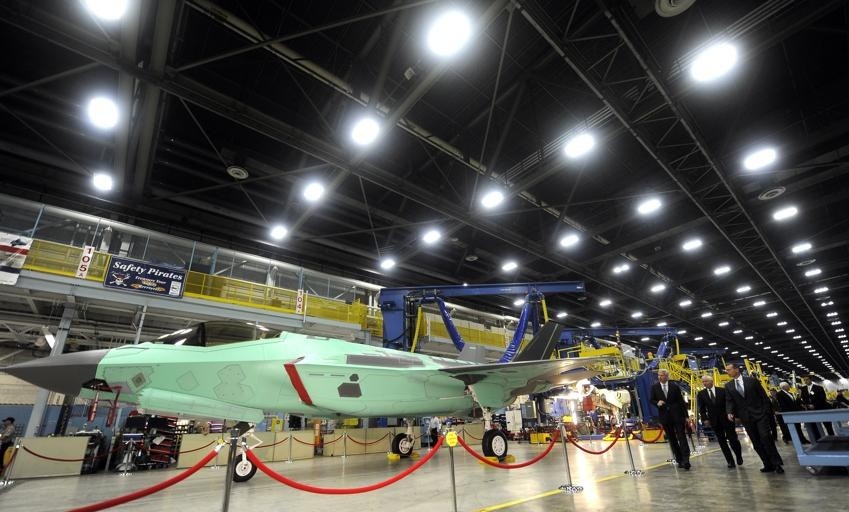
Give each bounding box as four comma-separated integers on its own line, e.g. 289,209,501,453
774,407,849,474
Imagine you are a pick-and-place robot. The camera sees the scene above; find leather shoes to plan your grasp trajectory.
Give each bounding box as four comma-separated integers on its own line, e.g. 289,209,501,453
677,462,691,471
736,457,743,465
727,461,736,469
759,466,776,472
775,465,785,475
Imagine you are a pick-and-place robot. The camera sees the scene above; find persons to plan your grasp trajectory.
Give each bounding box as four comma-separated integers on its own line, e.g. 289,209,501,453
724,361,785,475
406,416,417,443
697,374,744,467
1,416,16,474
650,367,691,469
768,374,849,445
426,415,441,448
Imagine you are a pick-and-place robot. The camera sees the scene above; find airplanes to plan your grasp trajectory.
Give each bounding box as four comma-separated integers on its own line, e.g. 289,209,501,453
1,314,610,490
10,238,27,247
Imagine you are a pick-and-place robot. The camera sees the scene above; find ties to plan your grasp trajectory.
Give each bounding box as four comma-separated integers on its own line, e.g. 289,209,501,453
708,389,716,403
735,379,745,398
662,385,668,400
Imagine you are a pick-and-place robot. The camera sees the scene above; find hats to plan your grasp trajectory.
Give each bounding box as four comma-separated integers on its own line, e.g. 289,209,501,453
2,416,15,422
